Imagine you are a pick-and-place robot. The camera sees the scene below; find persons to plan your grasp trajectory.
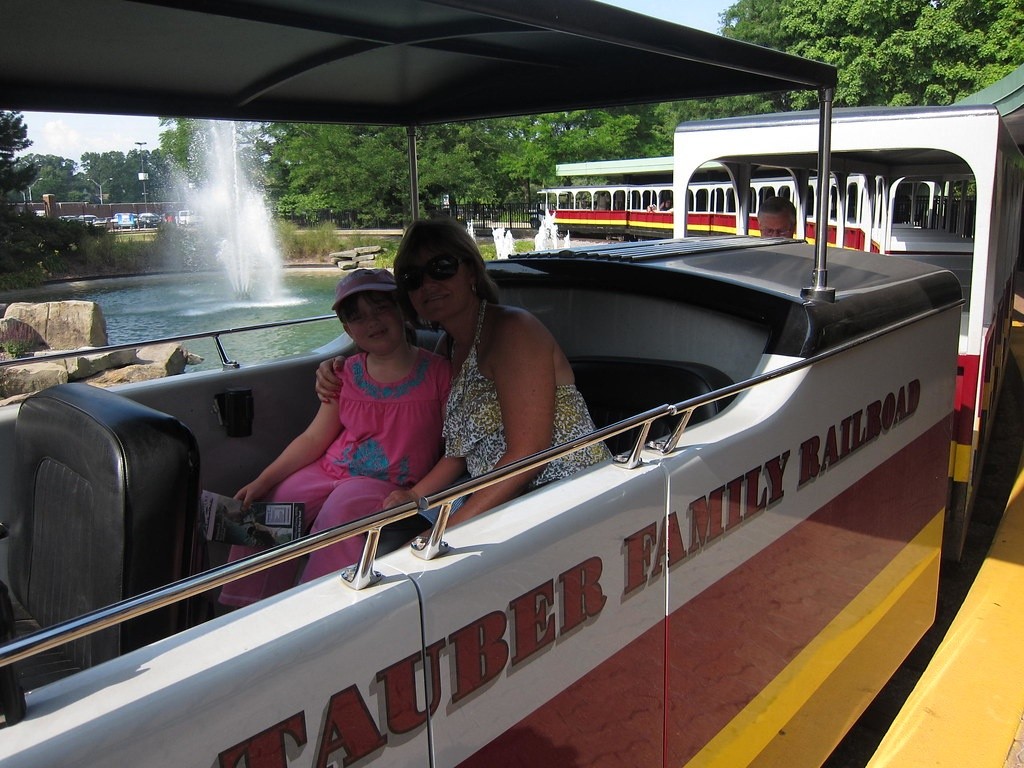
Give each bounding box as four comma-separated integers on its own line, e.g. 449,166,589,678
757,197,797,239
218,268,453,607
315,217,613,546
599,192,610,209
661,200,672,211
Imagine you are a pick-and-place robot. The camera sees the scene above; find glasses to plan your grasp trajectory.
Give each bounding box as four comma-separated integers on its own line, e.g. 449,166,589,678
400,254,461,291
759,224,794,236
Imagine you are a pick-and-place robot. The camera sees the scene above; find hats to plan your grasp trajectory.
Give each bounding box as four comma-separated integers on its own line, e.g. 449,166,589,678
331,269,397,310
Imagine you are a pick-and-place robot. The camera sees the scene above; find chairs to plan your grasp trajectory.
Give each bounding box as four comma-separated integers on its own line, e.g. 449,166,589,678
5,380,210,695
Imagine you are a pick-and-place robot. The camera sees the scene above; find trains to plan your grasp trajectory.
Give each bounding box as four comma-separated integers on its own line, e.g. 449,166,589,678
0,0,1024,768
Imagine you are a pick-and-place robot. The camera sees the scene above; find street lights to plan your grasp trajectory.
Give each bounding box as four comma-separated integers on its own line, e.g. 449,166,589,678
135,142,149,213
26,178,43,203
87,177,113,204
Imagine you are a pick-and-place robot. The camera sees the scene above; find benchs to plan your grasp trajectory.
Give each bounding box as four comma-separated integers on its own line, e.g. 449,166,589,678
566,357,737,453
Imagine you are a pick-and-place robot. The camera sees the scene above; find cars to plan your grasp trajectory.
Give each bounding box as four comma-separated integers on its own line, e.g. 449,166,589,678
59,210,200,229
